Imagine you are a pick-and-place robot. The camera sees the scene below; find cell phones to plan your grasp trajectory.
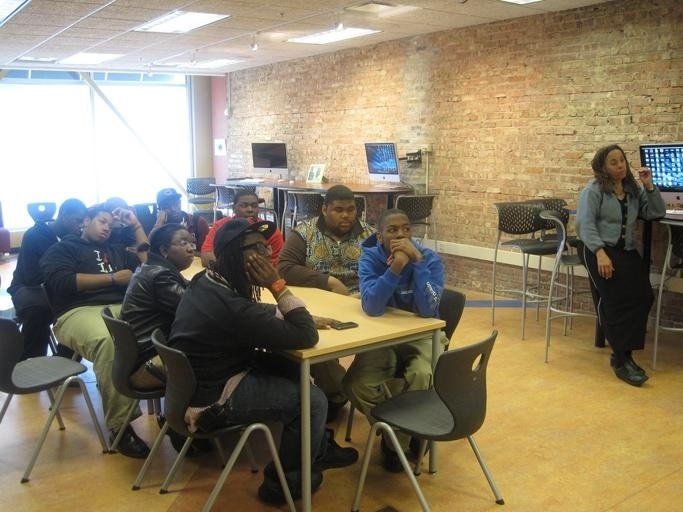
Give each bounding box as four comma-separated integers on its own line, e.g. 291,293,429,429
335,320,358,331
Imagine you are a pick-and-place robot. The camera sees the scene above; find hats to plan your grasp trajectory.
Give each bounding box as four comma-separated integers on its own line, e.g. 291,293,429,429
154,187,182,206
209,216,278,260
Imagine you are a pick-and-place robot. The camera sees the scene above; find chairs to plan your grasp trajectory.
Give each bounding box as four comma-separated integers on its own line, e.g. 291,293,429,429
181,177,439,261
348,328,506,511
0,277,112,485
97,307,169,493
343,287,469,460
480,190,683,373
26,202,57,229
149,326,298,511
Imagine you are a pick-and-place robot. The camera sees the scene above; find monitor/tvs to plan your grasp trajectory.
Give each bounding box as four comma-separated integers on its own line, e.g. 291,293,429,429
251,142,289,182
638,143,683,214
365,142,403,189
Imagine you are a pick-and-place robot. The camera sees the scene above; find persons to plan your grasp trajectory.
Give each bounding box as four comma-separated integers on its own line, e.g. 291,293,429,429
574,146,666,387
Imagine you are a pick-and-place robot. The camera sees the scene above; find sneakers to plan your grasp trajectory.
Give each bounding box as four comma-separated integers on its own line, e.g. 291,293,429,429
326,398,349,422
57,375,80,388
380,437,402,474
404,436,430,464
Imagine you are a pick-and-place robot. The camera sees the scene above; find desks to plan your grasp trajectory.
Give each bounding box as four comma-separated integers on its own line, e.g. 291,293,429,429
165,252,449,511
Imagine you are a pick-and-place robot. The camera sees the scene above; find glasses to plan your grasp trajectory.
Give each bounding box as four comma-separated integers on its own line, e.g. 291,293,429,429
234,238,275,258
167,238,197,251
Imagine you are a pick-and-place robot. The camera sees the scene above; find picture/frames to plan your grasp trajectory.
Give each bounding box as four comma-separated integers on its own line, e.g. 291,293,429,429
305,163,325,184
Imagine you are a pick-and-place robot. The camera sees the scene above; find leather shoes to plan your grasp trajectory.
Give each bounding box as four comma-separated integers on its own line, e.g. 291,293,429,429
155,414,195,457
609,353,645,375
311,428,359,470
107,422,150,459
612,361,650,387
258,470,324,504
